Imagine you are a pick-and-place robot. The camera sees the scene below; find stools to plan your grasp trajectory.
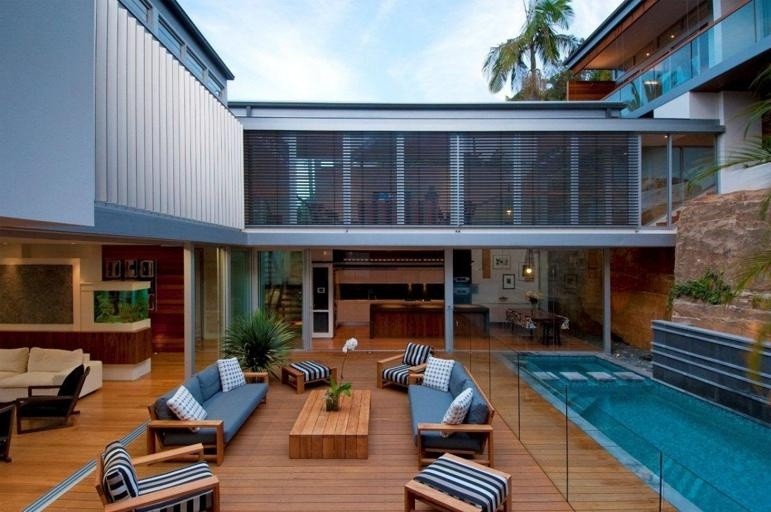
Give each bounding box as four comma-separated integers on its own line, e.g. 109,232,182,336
283,358,338,394
405,451,514,511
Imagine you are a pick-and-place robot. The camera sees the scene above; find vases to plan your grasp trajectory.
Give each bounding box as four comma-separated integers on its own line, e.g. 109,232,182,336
531,303,538,312
326,392,341,410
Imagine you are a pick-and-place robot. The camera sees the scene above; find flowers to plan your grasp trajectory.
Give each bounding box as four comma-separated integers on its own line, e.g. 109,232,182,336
525,290,546,304
324,337,359,398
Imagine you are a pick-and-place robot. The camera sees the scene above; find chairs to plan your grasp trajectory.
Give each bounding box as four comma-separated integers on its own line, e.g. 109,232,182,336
376,342,440,393
96,439,221,511
1,403,18,463
505,307,569,346
17,364,92,432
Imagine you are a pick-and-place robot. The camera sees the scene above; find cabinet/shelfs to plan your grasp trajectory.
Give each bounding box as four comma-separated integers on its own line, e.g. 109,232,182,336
312,261,335,338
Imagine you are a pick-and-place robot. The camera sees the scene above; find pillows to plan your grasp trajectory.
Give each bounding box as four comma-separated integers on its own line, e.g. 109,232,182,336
422,356,456,392
216,356,248,392
440,387,474,439
166,384,209,433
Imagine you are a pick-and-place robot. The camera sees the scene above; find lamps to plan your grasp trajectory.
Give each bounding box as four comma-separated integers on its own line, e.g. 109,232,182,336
526,248,533,274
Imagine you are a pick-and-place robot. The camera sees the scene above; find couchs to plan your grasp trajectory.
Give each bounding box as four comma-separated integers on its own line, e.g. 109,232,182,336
148,356,270,467
0,344,104,402
407,355,496,471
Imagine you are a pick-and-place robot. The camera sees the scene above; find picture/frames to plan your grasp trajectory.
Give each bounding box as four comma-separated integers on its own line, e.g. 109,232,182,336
493,254,512,270
564,273,579,289
503,273,516,289
